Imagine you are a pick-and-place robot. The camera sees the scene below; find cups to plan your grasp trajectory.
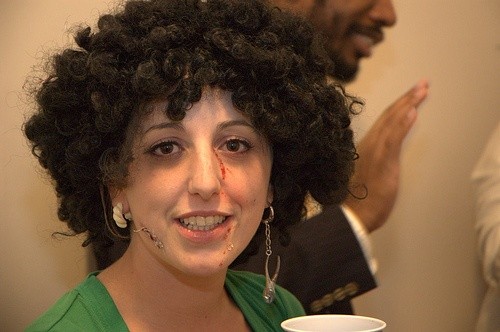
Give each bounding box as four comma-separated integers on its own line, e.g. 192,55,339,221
280,314,386,332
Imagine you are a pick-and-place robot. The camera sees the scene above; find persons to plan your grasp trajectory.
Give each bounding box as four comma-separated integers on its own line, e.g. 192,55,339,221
468,117,500,332
228,0,433,321
15,0,365,332
